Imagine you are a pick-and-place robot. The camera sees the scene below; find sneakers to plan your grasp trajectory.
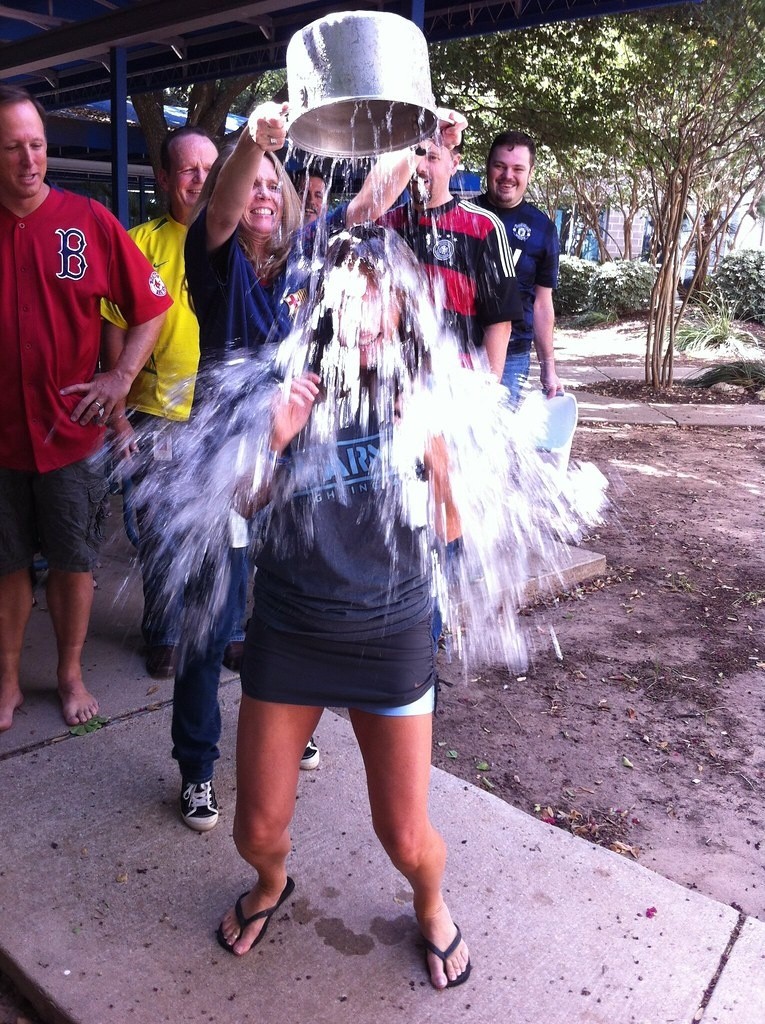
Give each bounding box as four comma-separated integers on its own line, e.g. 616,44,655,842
180,776,219,831
299,735,320,770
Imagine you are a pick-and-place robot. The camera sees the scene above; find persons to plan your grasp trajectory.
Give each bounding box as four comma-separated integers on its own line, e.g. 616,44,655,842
106,132,250,682
463,133,564,407
217,223,471,994
371,141,515,555
0,87,173,729
168,102,466,831
291,166,328,228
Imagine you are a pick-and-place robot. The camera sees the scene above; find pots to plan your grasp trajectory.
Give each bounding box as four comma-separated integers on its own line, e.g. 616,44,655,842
275,12,456,157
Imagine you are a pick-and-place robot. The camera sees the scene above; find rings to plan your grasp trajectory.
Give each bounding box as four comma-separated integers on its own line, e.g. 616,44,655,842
270,137,277,145
94,402,102,408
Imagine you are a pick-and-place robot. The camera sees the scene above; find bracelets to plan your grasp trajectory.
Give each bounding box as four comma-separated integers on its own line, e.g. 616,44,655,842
409,143,431,157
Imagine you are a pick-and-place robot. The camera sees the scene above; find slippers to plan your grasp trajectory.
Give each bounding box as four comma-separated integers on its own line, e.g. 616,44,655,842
414,923,470,987
216,876,295,954
